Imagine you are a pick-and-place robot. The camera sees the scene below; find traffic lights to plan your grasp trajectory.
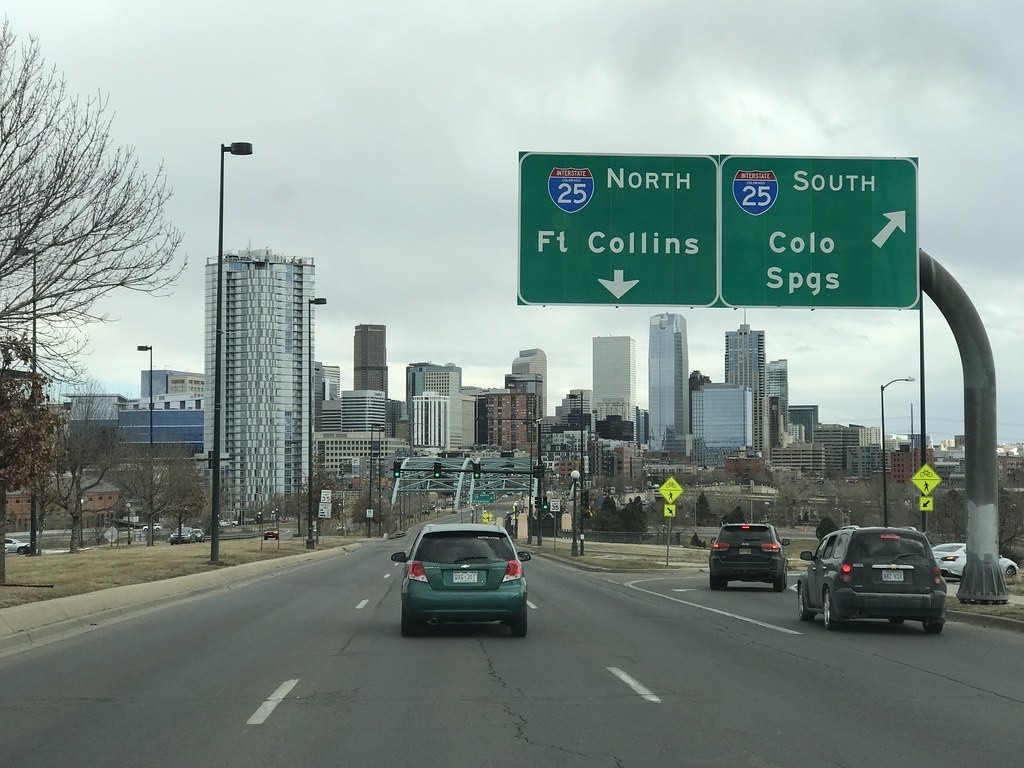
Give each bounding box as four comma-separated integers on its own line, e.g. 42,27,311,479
543,496,548,509
393,461,400,478
434,463,441,478
474,464,480,478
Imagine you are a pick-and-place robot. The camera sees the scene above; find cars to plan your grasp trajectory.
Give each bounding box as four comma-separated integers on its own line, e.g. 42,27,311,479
384,522,532,639
932,542,1019,580
219,519,238,528
263,527,279,540
4,538,30,555
169,526,205,545
143,523,163,531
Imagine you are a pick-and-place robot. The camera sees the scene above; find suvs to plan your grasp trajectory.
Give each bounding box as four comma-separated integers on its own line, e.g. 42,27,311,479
709,520,789,593
793,524,947,634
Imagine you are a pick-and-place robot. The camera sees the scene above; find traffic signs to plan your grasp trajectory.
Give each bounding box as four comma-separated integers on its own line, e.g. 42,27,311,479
473,493,494,504
517,147,923,314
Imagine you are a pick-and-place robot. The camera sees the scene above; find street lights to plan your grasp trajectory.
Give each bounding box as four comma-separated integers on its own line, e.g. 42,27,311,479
570,470,581,557
879,375,915,531
569,390,585,556
136,344,156,548
305,297,328,551
209,140,255,571
10,245,39,558
366,423,387,540
